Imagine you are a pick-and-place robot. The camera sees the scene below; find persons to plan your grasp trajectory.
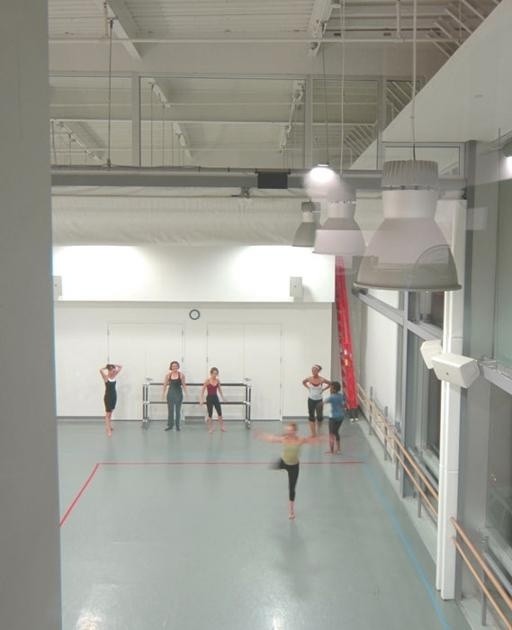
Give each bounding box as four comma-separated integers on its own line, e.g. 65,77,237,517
200,367,226,434
99,363,123,437
163,361,188,431
316,381,350,455
303,364,331,437
252,422,334,520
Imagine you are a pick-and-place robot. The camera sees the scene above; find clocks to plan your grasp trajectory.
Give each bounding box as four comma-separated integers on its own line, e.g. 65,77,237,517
189,309,200,320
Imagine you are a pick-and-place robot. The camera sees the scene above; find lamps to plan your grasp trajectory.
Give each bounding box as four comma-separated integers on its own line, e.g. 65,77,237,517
313,1,367,255
292,200,322,248
354,1,461,289
306,28,348,203
